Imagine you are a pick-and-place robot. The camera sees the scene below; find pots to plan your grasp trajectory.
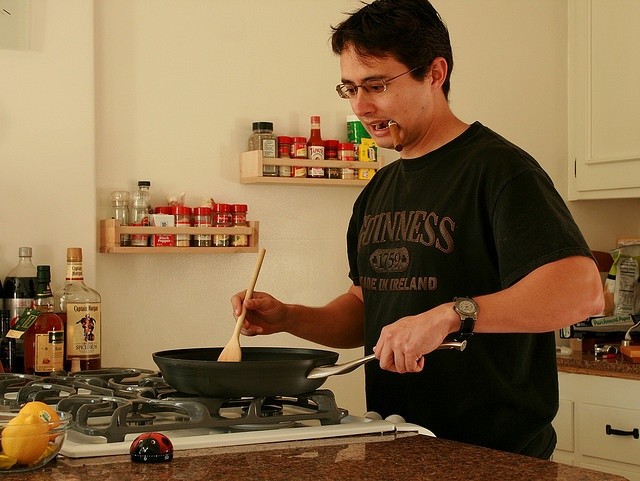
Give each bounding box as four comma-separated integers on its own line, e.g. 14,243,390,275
153,339,467,398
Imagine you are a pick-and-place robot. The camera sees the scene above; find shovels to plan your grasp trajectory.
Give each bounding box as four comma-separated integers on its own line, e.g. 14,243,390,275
217,248,266,362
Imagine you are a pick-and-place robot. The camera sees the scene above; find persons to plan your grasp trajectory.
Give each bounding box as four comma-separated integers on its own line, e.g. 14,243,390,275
230,2,606,458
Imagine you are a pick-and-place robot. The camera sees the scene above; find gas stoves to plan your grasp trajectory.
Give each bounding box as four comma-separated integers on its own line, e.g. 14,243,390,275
3,367,394,457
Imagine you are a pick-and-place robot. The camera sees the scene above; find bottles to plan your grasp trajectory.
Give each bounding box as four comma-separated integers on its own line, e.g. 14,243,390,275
0,278,4,371
155,207,170,215
232,204,249,247
23,265,65,376
55,248,101,371
278,136,291,177
212,204,232,246
111,192,129,245
130,191,150,246
247,121,278,176
173,206,192,246
308,116,326,177
4,246,37,373
138,181,150,211
194,207,212,246
338,142,357,180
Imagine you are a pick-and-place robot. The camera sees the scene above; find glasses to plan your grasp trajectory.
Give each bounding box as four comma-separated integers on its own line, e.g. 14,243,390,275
335,64,425,100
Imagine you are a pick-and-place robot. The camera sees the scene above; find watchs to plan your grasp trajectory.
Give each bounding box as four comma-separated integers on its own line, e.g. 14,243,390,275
452,295,480,343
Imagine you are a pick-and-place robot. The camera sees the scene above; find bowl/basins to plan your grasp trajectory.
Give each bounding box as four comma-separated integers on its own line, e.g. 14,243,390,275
1,409,72,473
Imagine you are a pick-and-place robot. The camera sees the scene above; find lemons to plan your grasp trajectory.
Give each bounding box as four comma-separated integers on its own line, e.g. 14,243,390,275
18,401,61,443
1,411,51,462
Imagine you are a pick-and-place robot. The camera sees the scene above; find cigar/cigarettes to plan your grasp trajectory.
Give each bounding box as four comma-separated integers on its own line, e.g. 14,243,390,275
388,120,405,153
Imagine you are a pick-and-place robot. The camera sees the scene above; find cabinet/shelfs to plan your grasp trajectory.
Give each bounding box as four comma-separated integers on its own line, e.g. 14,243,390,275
567,0,639,201
552,364,640,481
